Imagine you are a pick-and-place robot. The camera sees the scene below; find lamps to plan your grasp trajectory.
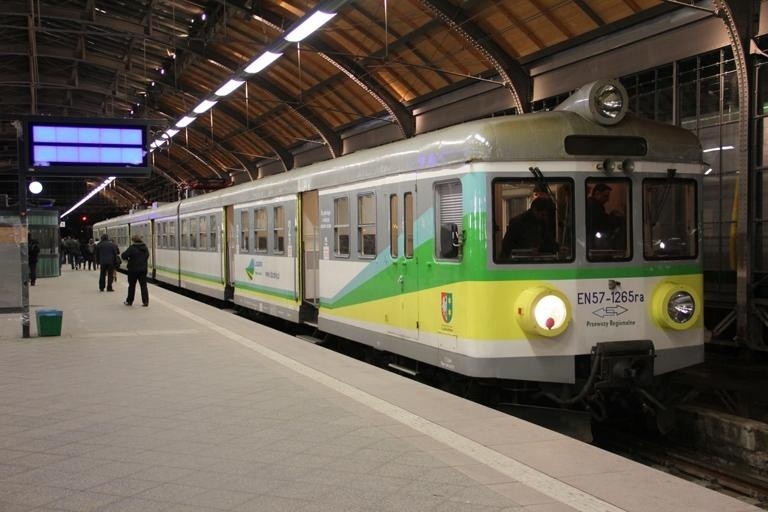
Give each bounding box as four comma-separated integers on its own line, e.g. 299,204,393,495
27,175,43,198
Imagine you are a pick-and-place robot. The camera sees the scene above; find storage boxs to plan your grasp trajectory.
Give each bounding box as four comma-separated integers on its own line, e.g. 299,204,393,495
34,308,64,338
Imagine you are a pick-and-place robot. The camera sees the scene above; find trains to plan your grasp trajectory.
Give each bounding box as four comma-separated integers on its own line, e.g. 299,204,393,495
89,75,712,395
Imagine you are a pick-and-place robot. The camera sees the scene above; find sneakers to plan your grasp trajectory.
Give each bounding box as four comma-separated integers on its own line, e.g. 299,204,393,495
124,301,148,307
100,288,115,291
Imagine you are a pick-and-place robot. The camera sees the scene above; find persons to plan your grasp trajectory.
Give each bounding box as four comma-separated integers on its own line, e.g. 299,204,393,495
60,233,122,282
27,231,40,285
121,233,149,307
531,185,549,202
500,195,568,259
95,234,115,292
586,183,625,247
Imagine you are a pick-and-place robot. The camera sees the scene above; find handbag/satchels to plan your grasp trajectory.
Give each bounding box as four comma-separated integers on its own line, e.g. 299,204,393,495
115,256,121,267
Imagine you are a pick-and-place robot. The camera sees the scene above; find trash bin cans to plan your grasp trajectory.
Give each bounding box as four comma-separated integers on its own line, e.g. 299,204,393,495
36,310,62,336
